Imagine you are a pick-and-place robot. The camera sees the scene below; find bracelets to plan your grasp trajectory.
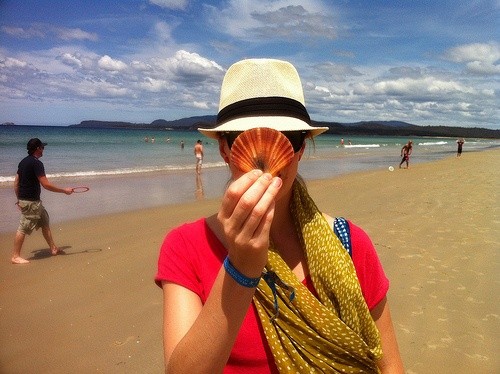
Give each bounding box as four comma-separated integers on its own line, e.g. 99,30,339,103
15,200,20,205
224,254,296,322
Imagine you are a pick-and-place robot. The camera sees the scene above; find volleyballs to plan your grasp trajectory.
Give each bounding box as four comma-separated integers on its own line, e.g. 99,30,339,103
389,165,395,171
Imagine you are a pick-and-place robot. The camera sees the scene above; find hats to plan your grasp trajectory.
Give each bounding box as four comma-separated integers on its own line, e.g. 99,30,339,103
197,140,202,142
198,58,329,139
27,138,48,149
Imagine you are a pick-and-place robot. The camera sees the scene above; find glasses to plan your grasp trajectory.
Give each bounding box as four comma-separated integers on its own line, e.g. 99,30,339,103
220,132,307,153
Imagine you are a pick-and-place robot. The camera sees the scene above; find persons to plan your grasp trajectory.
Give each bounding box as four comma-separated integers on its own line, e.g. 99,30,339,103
194,139,204,175
341,137,344,146
456,138,464,157
399,140,412,169
153,57,407,374
180,139,185,153
11,138,74,264
348,137,351,145
